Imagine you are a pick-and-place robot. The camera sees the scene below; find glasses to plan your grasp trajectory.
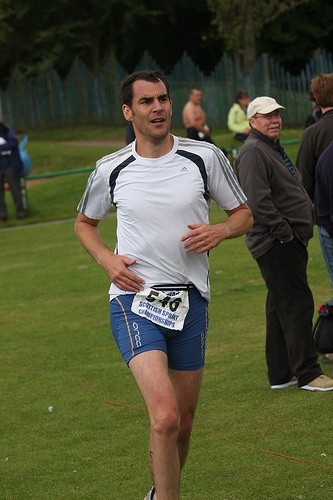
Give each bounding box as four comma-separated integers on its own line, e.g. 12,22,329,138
252,112,282,120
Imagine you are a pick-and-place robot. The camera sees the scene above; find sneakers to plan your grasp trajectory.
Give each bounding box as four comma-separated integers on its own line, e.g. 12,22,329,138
301,375,333,391
271,376,298,389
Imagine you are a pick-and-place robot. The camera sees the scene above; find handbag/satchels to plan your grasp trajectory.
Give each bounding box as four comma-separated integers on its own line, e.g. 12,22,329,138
312,313,333,353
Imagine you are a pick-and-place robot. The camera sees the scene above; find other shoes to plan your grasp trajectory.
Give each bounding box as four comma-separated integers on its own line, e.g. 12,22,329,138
325,353,333,361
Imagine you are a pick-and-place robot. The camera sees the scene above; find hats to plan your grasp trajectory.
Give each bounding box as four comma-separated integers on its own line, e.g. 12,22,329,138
247,96,287,120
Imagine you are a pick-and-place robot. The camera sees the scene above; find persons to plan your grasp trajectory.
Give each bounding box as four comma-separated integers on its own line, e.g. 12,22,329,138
234,96,333,390
227,92,251,141
183,89,217,147
73,72,254,500
295,74,333,363
0,121,25,222
